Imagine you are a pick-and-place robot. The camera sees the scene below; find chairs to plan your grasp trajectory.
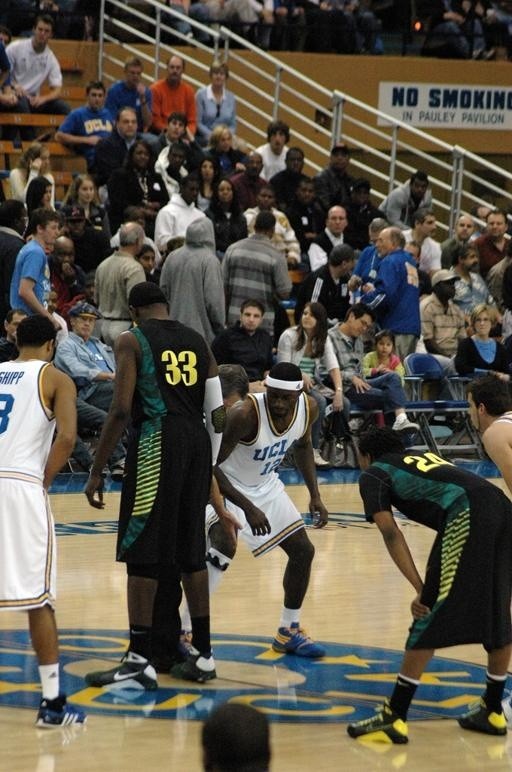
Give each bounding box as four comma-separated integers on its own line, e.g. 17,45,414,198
404,352,484,463
365,376,436,462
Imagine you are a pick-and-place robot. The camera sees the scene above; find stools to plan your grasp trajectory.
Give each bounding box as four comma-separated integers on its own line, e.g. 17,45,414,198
326,399,370,471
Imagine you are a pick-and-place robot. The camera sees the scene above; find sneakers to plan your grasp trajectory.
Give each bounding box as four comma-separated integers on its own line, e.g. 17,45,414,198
35,621,326,729
459,698,507,735
392,417,419,434
347,700,409,743
314,451,330,465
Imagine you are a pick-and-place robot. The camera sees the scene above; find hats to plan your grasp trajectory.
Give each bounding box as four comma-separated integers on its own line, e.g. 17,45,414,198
431,270,461,287
65,206,84,221
68,300,104,320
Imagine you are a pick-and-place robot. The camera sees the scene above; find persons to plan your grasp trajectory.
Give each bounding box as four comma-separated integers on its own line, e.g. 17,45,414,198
1,0,511,481
85,282,216,690
181,361,328,657
0,315,87,726
347,426,511,746
465,374,511,496
